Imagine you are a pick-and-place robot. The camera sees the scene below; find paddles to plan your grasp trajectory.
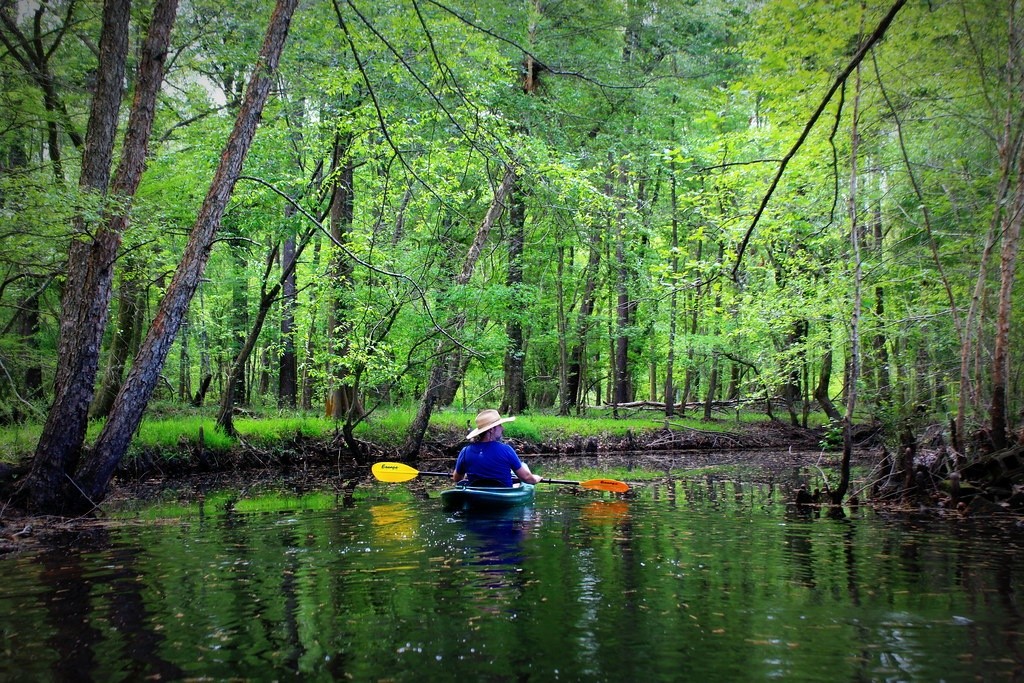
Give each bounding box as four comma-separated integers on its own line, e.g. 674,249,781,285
370,460,629,495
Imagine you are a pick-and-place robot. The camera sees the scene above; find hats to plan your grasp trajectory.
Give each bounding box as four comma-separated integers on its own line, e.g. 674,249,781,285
466,409,516,439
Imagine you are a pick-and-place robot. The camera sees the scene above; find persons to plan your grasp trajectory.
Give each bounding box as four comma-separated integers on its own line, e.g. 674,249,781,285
453,409,544,490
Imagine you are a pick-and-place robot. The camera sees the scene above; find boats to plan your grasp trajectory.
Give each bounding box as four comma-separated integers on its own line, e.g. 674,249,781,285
440,463,536,508
